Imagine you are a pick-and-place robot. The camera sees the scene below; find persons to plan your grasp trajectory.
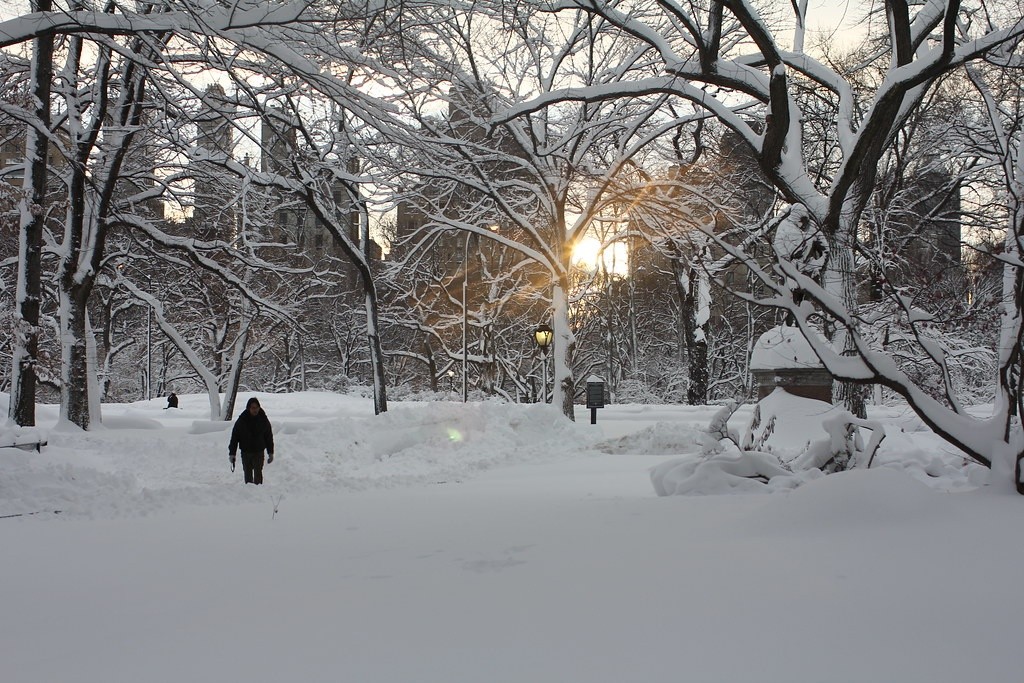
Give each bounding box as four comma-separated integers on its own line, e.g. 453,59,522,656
228,397,274,485
167,392,178,408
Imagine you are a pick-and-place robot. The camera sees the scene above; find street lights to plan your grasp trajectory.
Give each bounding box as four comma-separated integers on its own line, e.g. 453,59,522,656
462,218,500,402
116,257,151,400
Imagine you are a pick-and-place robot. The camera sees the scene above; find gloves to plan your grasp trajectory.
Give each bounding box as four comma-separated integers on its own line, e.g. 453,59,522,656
268,453,273,464
229,455,236,463
